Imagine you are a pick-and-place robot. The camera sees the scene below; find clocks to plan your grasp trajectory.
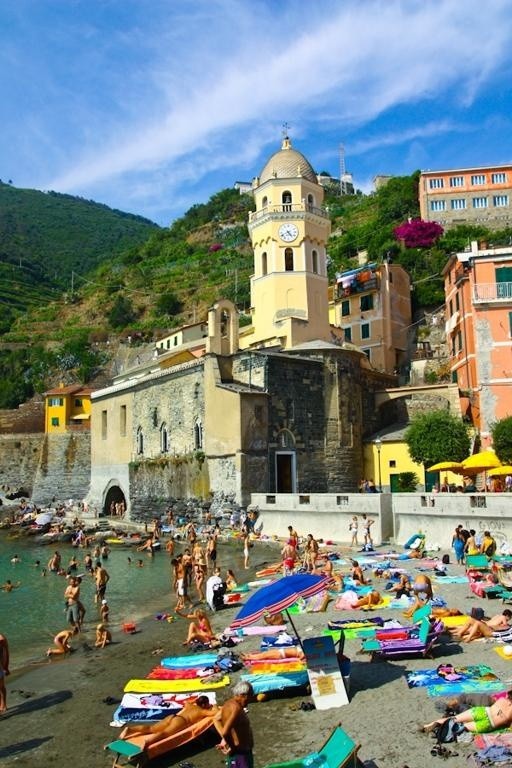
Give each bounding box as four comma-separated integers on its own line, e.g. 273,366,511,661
278,223,298,242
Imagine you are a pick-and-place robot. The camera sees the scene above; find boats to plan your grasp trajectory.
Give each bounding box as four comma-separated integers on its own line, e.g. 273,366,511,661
0,506,162,551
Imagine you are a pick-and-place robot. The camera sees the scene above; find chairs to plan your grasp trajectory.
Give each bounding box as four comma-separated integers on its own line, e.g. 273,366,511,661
239,629,350,697
356,603,445,662
104,705,223,768
465,554,512,605
263,720,361,768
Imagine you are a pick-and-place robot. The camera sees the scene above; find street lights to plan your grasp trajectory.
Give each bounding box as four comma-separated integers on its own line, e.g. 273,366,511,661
372,434,383,493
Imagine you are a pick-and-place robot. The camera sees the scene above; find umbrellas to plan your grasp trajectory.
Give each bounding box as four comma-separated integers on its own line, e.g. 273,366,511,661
462,451,502,492
486,465,512,492
427,461,462,477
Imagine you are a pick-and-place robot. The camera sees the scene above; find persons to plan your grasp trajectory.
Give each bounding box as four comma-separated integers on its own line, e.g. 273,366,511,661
1,475,511,767
